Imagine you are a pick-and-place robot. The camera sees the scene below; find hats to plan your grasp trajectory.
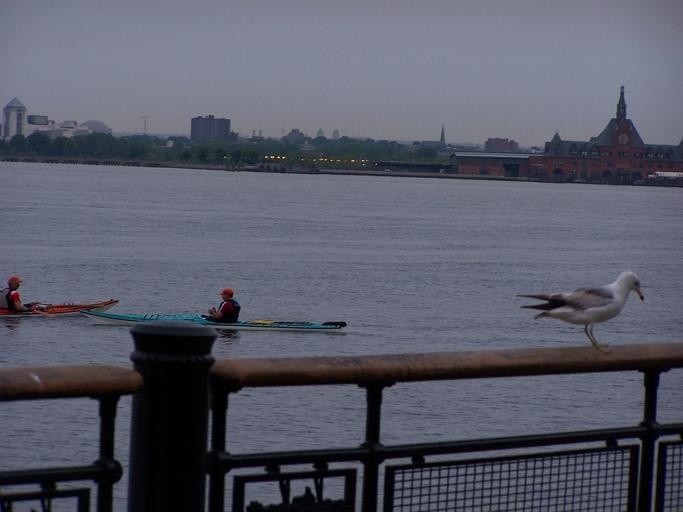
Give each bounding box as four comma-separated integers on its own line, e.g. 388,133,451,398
218,287,233,297
8,276,23,285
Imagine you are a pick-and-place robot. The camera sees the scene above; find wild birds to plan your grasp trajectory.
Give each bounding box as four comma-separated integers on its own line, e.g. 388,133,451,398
514,271,646,355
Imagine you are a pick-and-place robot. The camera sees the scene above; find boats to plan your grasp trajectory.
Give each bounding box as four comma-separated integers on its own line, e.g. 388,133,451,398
0,297,119,318
77,307,347,331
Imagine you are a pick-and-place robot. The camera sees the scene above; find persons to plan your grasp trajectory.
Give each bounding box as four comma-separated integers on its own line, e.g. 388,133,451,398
201,287,241,323
0,275,32,311
